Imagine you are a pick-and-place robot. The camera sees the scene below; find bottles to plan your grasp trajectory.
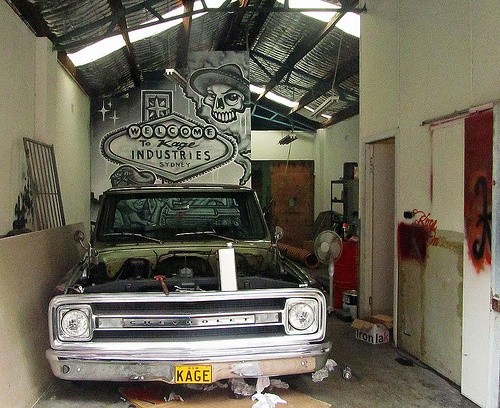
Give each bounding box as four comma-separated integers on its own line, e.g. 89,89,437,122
343,364,352,381
342,223,355,241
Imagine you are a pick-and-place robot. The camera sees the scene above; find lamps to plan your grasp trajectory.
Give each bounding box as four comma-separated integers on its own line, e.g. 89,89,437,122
309,30,339,118
278,116,298,144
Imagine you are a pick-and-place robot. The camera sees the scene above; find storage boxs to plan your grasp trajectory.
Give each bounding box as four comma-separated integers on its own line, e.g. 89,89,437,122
350,314,391,344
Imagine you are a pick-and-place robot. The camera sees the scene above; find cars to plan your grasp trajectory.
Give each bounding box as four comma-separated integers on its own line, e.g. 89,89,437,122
44,182,333,389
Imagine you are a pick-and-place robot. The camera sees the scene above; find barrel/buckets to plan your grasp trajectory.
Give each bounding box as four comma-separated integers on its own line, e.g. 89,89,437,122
342,291,358,319
333,239,360,308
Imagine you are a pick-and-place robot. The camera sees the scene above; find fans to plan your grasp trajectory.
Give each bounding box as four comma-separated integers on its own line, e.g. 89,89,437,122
314,230,342,314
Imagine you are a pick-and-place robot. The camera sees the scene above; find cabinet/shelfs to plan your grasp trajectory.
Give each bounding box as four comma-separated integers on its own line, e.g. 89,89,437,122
251,161,314,251
329,179,360,234
